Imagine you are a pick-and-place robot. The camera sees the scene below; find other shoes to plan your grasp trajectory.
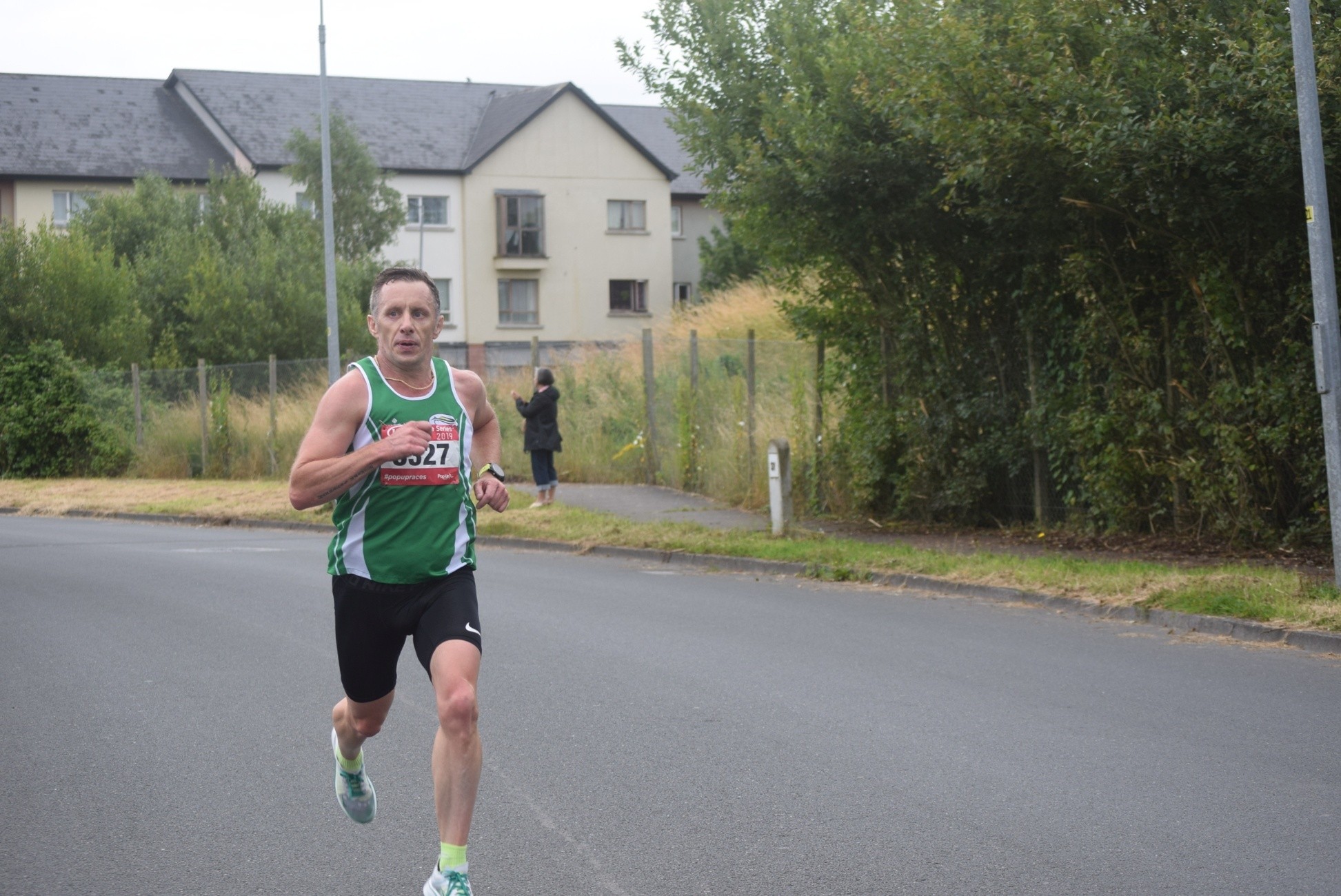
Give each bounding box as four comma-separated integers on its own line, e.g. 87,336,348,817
544,497,556,505
529,501,545,509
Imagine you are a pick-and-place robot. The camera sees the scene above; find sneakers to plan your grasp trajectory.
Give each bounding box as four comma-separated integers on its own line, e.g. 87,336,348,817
331,726,377,824
423,855,473,896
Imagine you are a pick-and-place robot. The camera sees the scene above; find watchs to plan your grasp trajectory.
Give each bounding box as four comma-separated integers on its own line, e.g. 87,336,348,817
479,463,505,481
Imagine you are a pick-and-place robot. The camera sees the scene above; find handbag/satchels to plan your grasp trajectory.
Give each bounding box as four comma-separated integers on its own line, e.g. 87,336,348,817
556,433,563,453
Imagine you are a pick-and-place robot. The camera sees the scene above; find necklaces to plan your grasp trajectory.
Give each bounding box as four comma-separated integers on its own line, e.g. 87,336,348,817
375,355,434,390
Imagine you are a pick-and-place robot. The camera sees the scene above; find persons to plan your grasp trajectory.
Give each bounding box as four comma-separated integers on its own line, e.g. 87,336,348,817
288,270,511,895
512,367,563,508
